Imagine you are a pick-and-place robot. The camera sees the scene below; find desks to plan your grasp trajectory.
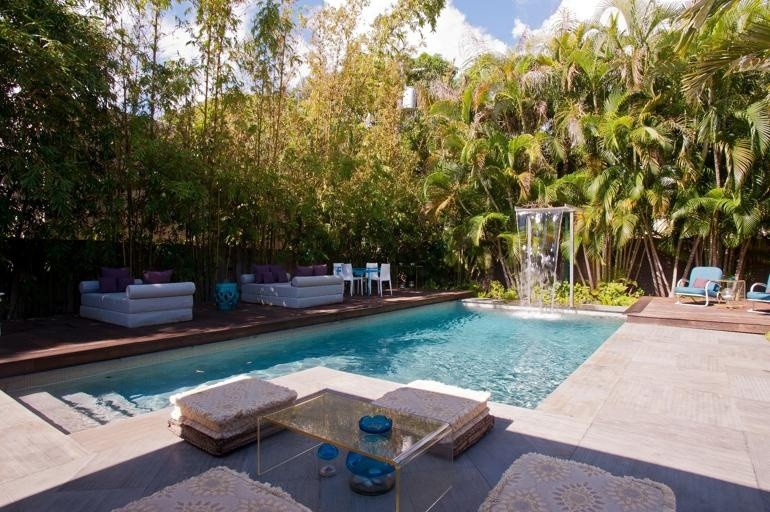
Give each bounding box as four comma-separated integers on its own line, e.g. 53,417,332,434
714,279,746,309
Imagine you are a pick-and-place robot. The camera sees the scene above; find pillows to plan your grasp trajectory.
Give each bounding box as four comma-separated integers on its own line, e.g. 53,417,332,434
254,264,274,283
295,264,313,277
273,266,287,282
97,266,131,293
140,268,175,284
314,263,328,276
116,275,133,293
263,271,274,283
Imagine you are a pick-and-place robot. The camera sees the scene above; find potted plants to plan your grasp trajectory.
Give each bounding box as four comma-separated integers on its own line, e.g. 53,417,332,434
214,220,243,311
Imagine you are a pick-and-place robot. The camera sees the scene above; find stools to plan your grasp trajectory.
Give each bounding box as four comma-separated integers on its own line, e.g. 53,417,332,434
163,372,301,463
112,464,327,512
370,380,493,459
478,452,677,512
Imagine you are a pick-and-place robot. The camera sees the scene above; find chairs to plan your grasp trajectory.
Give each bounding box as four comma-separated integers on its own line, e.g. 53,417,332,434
746,272,770,314
670,261,722,307
330,262,396,299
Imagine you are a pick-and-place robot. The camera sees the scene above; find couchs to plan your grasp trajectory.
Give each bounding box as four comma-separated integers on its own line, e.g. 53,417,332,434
79,278,196,329
239,271,345,308
